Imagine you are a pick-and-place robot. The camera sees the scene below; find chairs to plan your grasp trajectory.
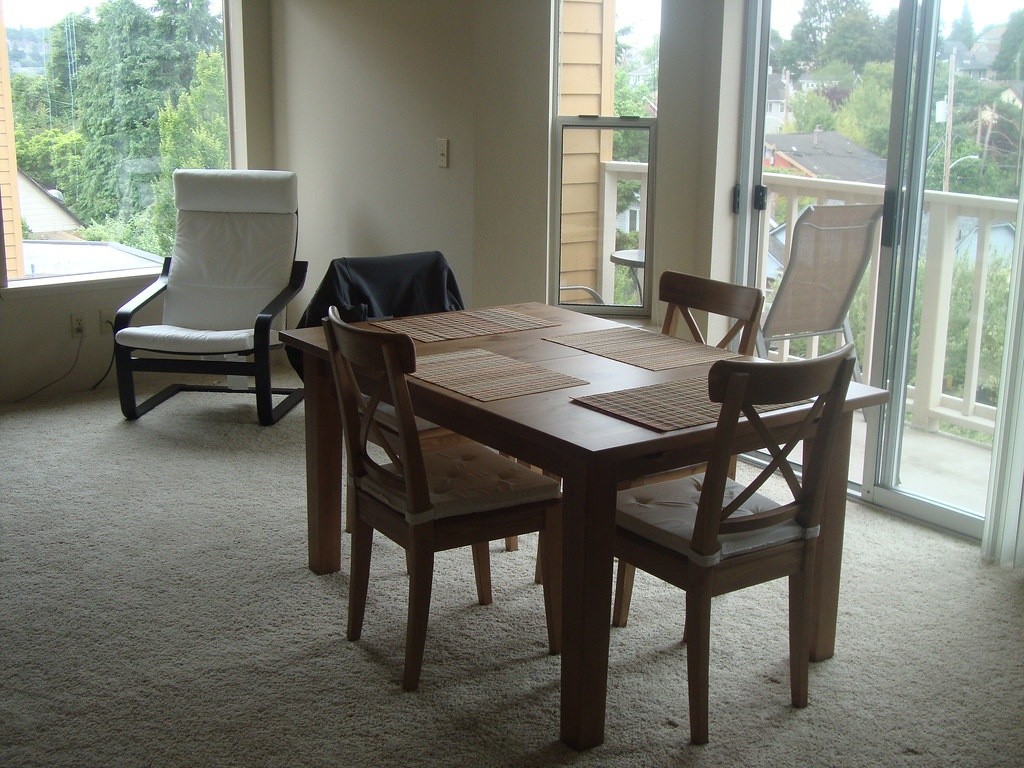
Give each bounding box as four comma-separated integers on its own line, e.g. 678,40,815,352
613,269,767,628
606,340,860,748
323,304,562,692
111,167,307,426
746,201,883,424
278,247,521,573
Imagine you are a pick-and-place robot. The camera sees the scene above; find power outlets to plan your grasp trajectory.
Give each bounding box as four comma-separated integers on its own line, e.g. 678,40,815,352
99,310,114,333
72,311,86,339
437,138,448,167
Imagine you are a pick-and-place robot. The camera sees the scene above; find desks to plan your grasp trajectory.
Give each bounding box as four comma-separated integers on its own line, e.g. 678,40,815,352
274,301,889,752
612,251,647,306
